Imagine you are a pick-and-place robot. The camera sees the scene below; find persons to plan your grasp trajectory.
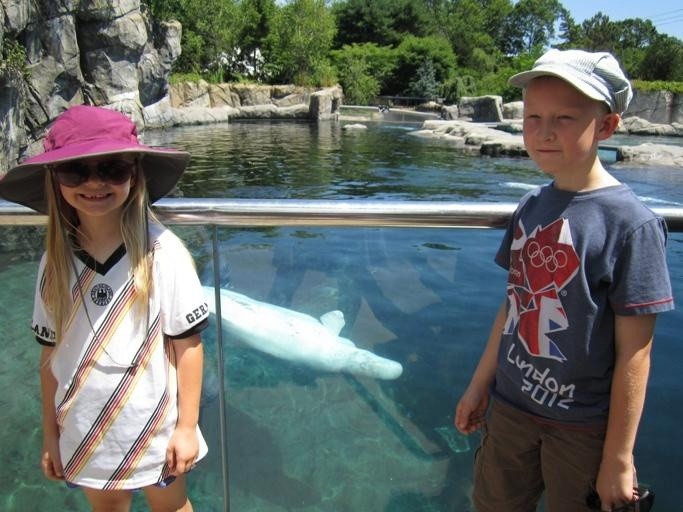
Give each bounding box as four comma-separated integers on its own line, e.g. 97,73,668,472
454,50,675,512
0,104,209,512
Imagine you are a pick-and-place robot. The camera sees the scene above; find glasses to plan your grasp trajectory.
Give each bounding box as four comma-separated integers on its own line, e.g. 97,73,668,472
51,158,138,189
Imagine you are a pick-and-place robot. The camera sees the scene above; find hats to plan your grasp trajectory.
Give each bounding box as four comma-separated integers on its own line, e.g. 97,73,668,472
0,106,192,216
508,48,634,115
